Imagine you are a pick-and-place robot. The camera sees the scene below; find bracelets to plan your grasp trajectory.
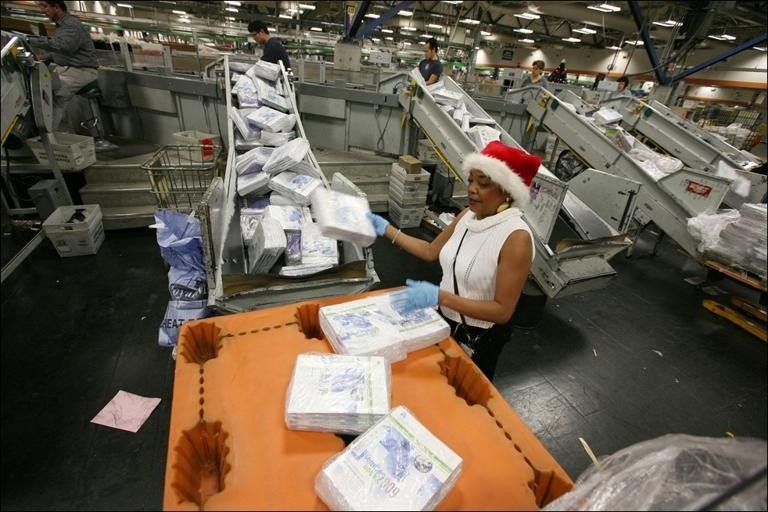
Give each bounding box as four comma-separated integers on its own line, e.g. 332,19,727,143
391,228,402,244
26,36,30,43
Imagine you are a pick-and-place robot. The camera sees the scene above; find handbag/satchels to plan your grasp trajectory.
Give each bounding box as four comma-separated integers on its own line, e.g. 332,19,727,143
453,323,498,377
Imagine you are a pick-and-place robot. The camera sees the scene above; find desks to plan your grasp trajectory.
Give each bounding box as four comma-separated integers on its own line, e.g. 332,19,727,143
163,286,591,512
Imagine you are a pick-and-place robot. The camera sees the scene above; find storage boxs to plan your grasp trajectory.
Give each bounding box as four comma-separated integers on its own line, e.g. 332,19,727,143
42,203,99,230
388,155,432,229
25,132,97,172
172,130,221,162
45,211,106,258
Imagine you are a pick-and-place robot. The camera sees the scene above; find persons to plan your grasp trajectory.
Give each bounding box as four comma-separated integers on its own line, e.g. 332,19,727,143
521,59,549,90
11,0,99,135
366,139,543,381
419,37,443,86
616,76,632,96
247,20,292,73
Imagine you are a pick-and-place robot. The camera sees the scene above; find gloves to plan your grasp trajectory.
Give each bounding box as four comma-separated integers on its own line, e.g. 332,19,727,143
368,213,391,237
392,277,440,316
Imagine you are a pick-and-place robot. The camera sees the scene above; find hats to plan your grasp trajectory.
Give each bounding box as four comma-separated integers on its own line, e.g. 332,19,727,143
462,139,542,209
